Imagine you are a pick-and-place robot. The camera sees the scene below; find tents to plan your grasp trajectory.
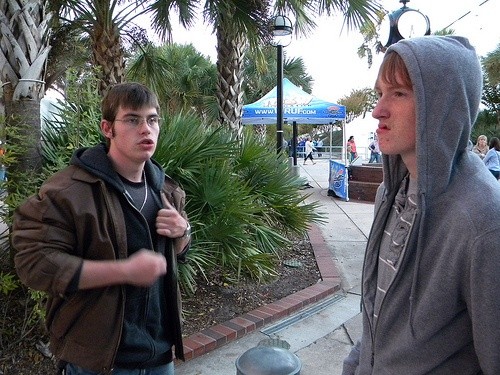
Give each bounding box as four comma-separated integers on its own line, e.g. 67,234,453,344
238,78,350,203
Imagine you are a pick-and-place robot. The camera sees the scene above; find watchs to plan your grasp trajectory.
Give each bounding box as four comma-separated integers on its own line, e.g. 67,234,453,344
175,220,192,239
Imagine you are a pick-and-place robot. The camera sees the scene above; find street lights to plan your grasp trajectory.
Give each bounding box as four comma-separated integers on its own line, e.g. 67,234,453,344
264,8,294,176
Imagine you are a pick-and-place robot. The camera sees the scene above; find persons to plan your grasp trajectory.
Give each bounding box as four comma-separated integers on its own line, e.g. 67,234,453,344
367,136,384,163
347,134,358,163
483,137,500,178
305,137,317,166
11,81,193,375
342,34,500,375
471,135,489,157
316,139,324,158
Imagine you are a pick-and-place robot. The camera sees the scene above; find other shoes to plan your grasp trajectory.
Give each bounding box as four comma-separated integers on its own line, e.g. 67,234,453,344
303,164,307,165
313,162,316,165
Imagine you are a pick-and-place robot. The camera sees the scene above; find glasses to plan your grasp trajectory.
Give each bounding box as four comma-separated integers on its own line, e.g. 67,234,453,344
108,117,164,128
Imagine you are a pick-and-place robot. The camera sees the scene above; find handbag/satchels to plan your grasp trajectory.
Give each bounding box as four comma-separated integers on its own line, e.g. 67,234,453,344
371,145,375,149
